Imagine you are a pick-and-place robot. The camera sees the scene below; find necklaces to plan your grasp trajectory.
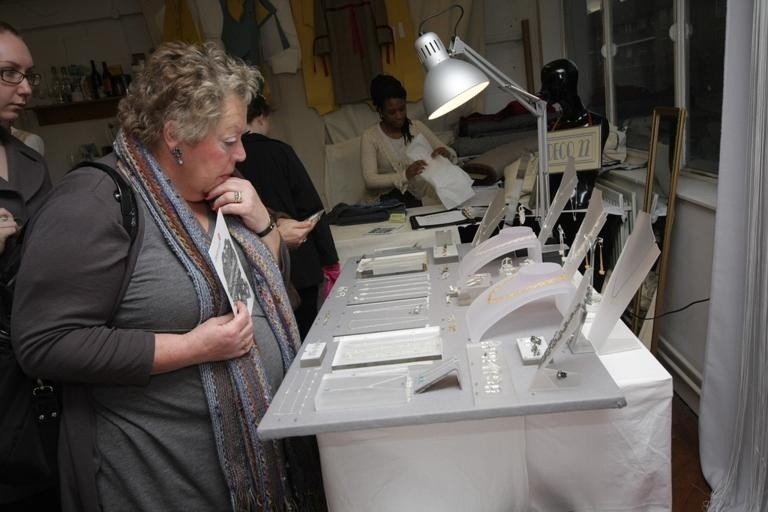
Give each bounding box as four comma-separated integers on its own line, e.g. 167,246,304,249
275,170,657,424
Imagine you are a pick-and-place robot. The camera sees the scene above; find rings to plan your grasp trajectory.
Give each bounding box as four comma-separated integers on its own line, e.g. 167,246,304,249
1,214,8,222
235,191,242,204
243,347,249,353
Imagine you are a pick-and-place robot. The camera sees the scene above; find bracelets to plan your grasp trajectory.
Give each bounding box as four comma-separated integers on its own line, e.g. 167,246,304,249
256,209,281,237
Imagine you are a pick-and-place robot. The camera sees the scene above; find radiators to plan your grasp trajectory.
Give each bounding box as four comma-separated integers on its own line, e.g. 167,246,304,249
596,179,636,270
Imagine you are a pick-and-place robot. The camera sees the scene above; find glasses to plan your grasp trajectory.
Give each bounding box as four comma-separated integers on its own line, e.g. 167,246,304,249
0,69,41,86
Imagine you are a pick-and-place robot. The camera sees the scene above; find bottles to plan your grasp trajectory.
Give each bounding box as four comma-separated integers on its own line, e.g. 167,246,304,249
31,60,133,107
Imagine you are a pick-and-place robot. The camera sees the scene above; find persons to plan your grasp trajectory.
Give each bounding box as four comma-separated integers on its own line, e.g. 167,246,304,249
230,168,320,252
539,57,609,227
234,92,341,344
360,74,457,209
1,22,54,510
11,37,322,512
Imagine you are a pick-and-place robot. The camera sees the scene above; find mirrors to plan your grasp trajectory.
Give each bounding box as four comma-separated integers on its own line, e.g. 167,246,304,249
631,105,687,356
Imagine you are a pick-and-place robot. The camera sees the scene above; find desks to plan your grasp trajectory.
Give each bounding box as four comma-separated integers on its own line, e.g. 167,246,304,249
316,270,674,511
329,203,462,269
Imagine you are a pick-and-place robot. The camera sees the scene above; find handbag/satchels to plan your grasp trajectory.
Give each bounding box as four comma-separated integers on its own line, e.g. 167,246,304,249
1,330,61,512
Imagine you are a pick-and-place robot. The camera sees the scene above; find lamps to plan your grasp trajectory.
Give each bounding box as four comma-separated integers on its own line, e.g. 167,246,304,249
414,5,551,224
